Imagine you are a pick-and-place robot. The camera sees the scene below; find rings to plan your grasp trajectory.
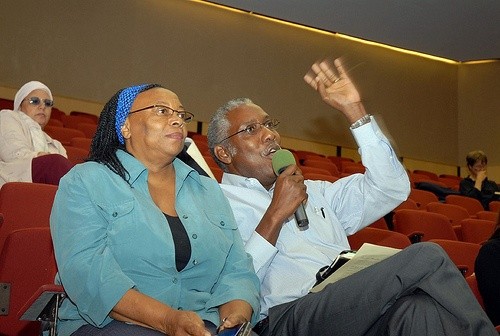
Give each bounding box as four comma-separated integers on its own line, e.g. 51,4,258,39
333,77,342,85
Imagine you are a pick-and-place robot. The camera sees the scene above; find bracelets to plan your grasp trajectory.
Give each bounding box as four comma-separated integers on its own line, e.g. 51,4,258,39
351,112,372,129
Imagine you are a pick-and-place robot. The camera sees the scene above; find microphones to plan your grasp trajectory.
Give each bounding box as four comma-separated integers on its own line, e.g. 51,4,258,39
272,149,309,228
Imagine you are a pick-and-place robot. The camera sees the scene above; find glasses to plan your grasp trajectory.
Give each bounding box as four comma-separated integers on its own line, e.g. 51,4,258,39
128,105,194,124
23,97,53,108
219,119,280,144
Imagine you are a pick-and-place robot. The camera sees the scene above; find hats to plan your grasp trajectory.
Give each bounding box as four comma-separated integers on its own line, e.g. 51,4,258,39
14,81,53,110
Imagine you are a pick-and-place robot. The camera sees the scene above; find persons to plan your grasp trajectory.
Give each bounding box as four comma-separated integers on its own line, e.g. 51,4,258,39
1,80,70,184
474,228,500,327
208,57,497,335
43,83,263,336
461,151,500,209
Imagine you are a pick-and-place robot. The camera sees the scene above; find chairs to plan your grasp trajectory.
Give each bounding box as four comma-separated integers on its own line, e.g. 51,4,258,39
0,99,500,336
